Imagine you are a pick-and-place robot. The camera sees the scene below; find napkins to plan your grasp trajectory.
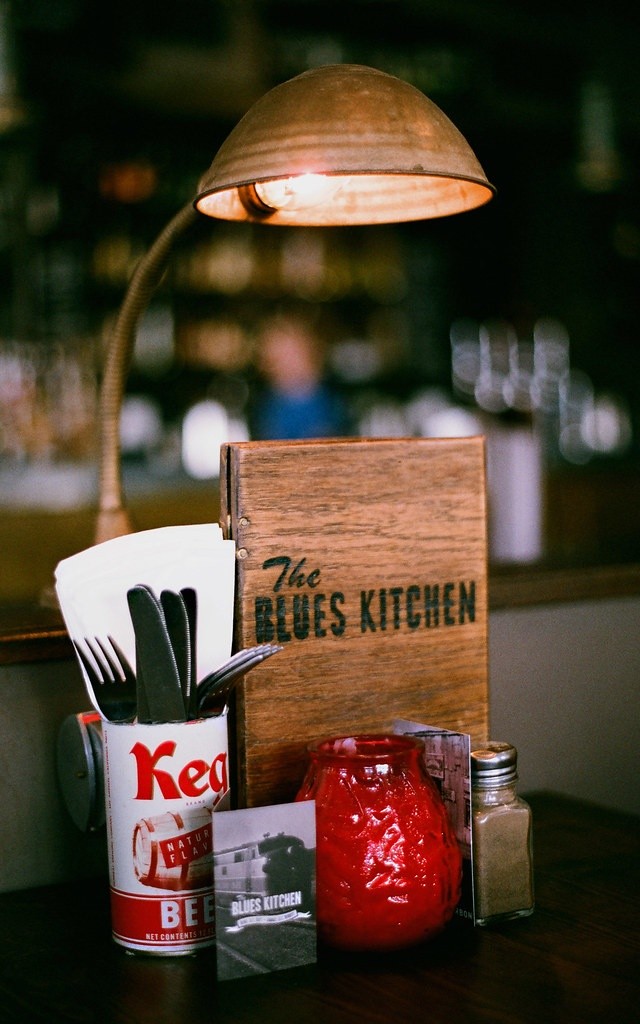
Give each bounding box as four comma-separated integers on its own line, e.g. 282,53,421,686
52,523,236,700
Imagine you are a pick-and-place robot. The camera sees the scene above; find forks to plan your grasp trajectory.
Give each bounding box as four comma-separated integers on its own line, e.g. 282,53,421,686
72,636,139,725
196,643,283,719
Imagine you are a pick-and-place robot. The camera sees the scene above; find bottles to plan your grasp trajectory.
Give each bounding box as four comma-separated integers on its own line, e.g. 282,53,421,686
471,745,535,927
296,730,463,954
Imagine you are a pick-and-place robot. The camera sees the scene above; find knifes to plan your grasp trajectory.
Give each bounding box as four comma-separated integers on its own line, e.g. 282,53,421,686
128,583,197,726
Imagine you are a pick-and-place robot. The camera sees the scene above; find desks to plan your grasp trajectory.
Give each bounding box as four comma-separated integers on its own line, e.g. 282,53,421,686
0,790,640,1024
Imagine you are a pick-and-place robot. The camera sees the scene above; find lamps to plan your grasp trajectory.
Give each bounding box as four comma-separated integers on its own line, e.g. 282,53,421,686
37,62,495,608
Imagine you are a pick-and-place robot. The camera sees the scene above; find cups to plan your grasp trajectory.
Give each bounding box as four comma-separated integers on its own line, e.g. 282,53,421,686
101,712,231,959
